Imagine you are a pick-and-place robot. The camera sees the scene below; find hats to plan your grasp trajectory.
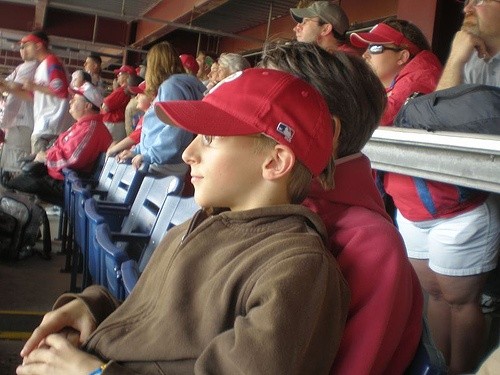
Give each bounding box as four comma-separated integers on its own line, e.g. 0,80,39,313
71,70,95,86
179,53,200,75
135,64,147,79
349,22,422,58
290,1,349,36
114,64,137,78
153,67,334,176
67,81,104,109
128,81,157,99
20,31,50,47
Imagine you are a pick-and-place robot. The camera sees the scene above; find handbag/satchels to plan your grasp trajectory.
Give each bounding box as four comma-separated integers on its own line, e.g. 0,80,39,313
393,82,500,136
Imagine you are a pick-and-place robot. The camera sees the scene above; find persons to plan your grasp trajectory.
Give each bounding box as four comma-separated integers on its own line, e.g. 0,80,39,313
0,0,500,375
22,29,69,159
350,18,500,375
7,82,113,262
16,68,352,375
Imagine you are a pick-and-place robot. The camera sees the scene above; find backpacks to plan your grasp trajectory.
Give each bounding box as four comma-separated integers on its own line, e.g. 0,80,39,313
0,187,52,263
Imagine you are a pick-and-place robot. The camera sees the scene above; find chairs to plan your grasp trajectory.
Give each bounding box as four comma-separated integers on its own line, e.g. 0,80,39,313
61,152,202,302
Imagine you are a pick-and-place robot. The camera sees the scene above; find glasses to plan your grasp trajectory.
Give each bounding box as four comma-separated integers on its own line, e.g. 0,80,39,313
300,18,321,25
367,44,403,55
201,134,262,146
464,0,500,7
